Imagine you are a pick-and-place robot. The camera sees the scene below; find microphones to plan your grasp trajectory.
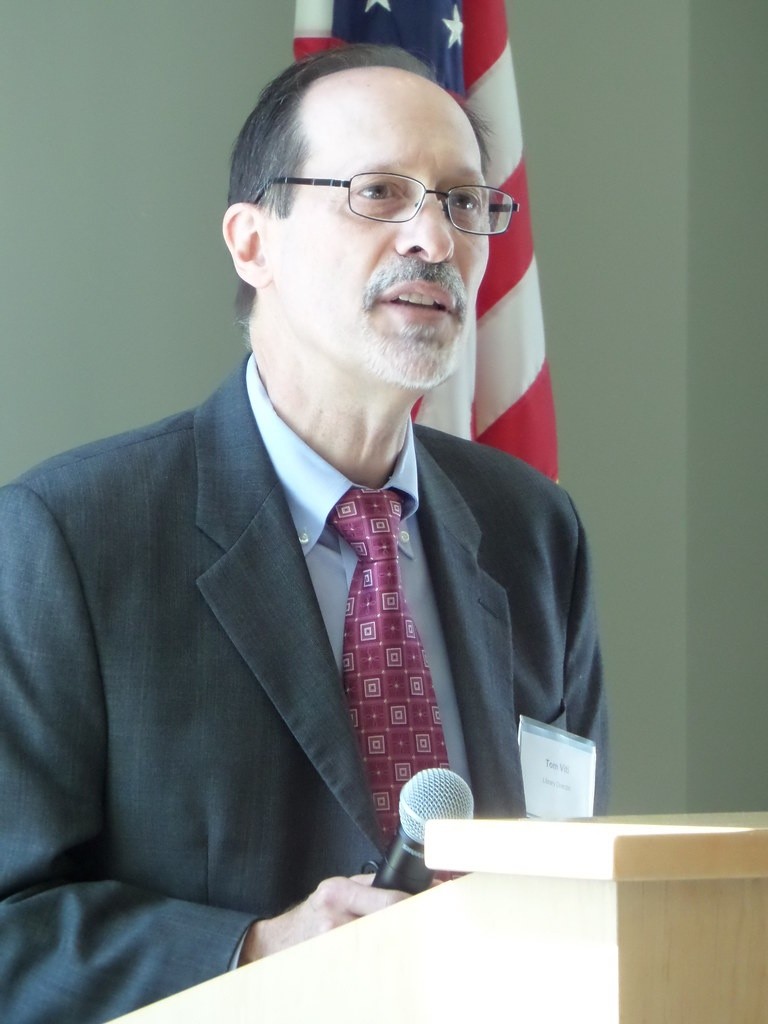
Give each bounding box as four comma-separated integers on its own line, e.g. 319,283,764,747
369,768,474,897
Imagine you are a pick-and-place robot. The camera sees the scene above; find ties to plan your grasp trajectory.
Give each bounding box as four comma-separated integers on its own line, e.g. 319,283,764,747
325,488,469,883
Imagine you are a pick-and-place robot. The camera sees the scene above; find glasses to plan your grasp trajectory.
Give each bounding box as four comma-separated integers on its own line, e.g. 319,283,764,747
255,173,520,237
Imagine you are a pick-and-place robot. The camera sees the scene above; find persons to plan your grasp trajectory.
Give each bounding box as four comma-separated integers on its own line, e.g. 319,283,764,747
0,42,614,1024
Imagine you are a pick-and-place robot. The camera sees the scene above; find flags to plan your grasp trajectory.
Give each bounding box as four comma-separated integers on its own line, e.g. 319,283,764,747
291,0,560,480
